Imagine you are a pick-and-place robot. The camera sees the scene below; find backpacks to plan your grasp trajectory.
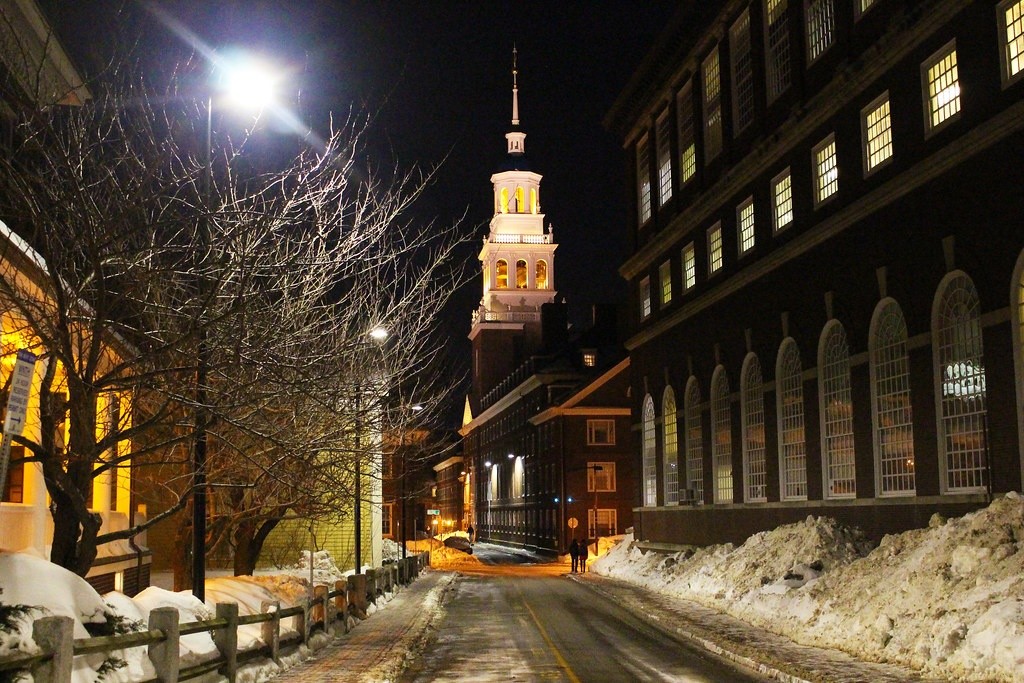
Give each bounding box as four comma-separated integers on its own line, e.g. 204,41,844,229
580,545,586,556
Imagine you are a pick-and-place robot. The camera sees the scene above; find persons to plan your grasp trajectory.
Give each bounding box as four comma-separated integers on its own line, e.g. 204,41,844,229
579,539,588,573
569,539,579,572
466,525,474,540
